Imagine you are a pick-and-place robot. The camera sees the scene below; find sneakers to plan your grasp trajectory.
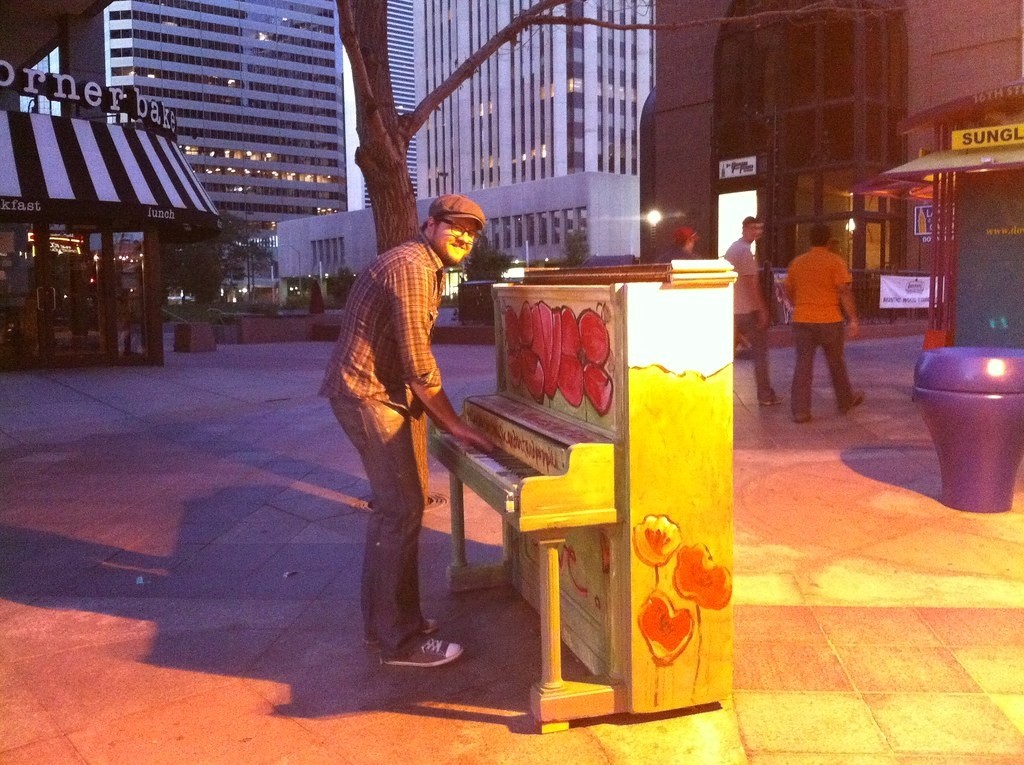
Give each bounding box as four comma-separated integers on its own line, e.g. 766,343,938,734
363,619,438,645
383,635,462,667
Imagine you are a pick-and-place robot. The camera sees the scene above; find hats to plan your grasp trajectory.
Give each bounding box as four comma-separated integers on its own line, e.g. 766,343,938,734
428,194,485,231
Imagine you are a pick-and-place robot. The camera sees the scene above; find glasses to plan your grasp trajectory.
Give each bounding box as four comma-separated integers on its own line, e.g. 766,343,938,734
435,216,480,244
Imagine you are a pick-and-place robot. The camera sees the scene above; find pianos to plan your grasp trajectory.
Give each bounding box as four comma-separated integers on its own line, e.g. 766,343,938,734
429,257,740,720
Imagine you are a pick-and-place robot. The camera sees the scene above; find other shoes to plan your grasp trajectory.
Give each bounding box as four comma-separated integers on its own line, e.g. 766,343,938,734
794,411,811,423
762,395,785,405
840,390,862,412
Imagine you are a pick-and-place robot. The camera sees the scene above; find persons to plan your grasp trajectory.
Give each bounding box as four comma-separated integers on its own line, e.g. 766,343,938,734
318,193,493,667
784,224,864,424
724,216,785,407
654,227,701,262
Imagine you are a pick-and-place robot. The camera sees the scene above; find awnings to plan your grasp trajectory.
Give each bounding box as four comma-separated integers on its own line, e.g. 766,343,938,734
0,110,225,243
